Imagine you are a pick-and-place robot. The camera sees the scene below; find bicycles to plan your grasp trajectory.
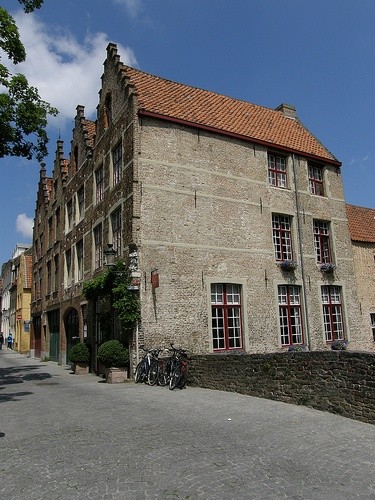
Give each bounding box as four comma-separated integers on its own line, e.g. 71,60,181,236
134,343,189,390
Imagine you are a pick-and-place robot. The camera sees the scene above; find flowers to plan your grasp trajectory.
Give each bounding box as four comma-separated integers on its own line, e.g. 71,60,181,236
279,259,292,266
332,337,349,350
321,263,335,273
289,344,309,352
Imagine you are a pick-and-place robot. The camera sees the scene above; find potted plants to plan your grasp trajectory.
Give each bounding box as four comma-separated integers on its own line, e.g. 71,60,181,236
69,343,90,375
97,340,130,384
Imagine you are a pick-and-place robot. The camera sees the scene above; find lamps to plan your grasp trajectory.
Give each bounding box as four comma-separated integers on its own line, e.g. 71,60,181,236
103,244,117,266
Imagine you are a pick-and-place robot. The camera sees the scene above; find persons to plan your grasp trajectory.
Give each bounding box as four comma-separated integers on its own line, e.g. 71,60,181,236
0,331,4,350
7,333,12,350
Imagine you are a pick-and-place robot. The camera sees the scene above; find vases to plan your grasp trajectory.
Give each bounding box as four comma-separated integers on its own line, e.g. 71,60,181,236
281,266,295,271
324,267,333,274
